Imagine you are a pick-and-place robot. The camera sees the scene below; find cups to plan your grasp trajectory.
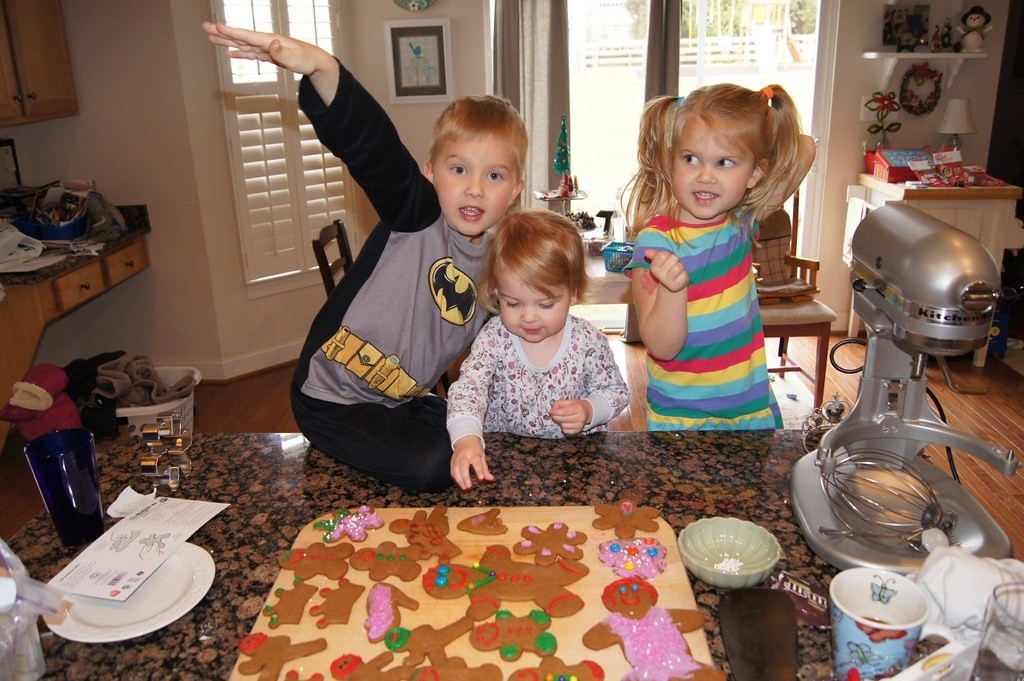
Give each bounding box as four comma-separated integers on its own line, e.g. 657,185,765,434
831,568,930,681
24,429,101,544
971,583,1024,681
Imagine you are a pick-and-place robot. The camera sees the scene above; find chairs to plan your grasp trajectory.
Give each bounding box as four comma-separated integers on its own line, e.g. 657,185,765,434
313,220,451,397
752,186,839,413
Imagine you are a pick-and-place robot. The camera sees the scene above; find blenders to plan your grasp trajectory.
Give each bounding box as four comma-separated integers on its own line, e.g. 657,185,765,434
789,206,1015,577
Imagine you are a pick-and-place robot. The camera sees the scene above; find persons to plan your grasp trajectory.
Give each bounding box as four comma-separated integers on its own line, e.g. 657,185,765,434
618,83,815,433
446,208,632,490
202,20,530,494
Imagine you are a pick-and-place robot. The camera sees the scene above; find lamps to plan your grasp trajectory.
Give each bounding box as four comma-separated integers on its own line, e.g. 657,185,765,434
938,97,978,166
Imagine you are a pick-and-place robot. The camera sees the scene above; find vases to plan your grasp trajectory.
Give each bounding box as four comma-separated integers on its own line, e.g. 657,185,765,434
865,150,877,174
873,132,890,152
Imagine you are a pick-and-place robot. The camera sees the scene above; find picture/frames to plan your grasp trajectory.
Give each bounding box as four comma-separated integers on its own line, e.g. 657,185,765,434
382,17,456,104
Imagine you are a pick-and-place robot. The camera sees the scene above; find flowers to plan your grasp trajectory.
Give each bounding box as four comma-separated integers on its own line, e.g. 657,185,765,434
864,91,903,148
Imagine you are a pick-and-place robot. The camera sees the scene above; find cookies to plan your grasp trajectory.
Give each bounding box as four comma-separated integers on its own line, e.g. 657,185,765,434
237,499,726,681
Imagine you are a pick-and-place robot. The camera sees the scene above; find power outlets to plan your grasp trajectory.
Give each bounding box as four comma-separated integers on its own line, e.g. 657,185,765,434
859,96,900,122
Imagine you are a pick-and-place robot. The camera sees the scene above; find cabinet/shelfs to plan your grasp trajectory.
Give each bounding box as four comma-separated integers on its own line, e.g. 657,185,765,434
0,0,81,128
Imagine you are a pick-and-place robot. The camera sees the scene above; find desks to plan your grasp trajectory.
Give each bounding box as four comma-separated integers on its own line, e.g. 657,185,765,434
0,432,1024,681
585,257,641,342
0,225,152,451
847,173,1023,367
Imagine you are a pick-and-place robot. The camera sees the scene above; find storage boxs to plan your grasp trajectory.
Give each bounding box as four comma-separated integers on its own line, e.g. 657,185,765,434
873,148,936,183
121,365,202,432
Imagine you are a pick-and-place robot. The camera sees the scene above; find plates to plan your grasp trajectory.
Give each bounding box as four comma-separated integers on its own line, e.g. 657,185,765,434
679,518,781,584
41,543,216,643
